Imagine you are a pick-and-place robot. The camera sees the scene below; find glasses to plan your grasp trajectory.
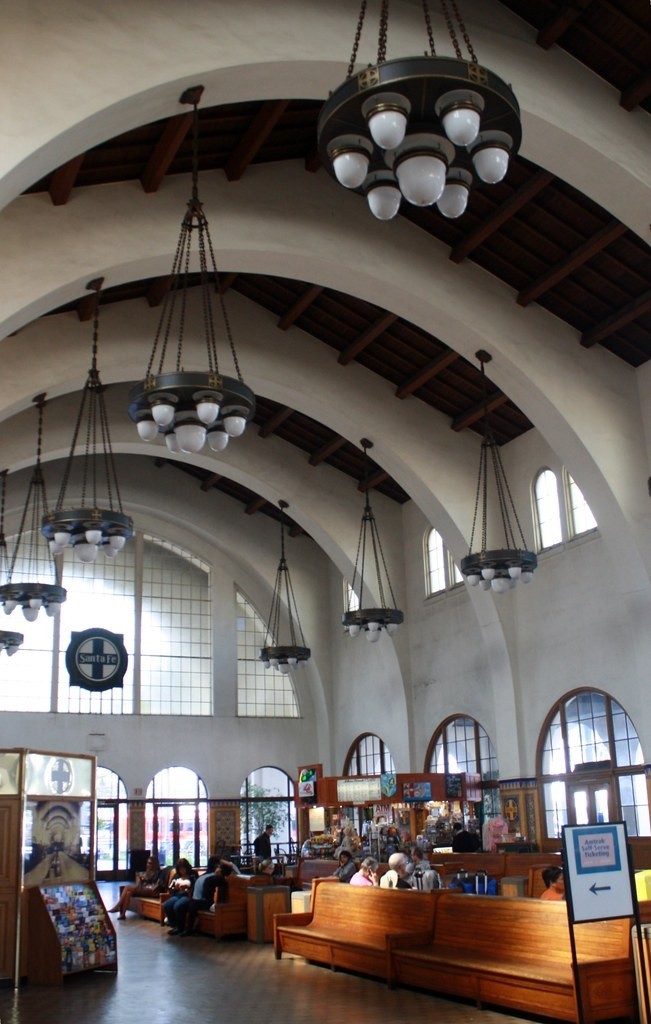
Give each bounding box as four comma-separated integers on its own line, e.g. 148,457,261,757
267,866,275,870
147,861,153,865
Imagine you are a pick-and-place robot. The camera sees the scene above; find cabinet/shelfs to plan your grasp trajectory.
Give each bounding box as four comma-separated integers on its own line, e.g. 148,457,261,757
27,880,119,987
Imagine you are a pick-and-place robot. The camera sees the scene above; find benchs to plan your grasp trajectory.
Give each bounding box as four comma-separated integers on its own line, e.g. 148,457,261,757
119,850,651,1024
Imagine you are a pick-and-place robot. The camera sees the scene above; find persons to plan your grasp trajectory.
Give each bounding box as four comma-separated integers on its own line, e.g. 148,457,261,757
108,856,160,920
541,866,564,901
452,822,477,853
161,858,231,936
333,847,429,890
254,825,274,882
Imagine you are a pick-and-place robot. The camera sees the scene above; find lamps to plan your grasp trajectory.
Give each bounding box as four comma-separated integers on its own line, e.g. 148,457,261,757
40,276,134,563
0,392,67,622
0,469,23,657
342,439,404,641
317,0,524,220
460,348,537,594
129,83,258,457
259,499,310,675
